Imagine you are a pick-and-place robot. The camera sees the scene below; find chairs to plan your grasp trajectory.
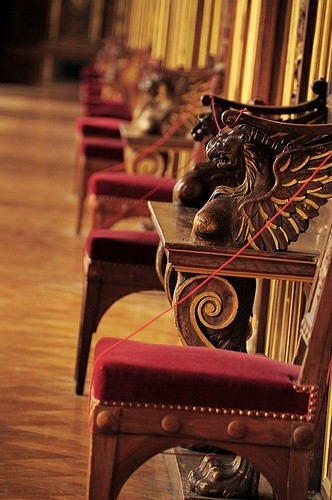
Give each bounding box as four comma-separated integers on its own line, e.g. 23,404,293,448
76,44,332,500
